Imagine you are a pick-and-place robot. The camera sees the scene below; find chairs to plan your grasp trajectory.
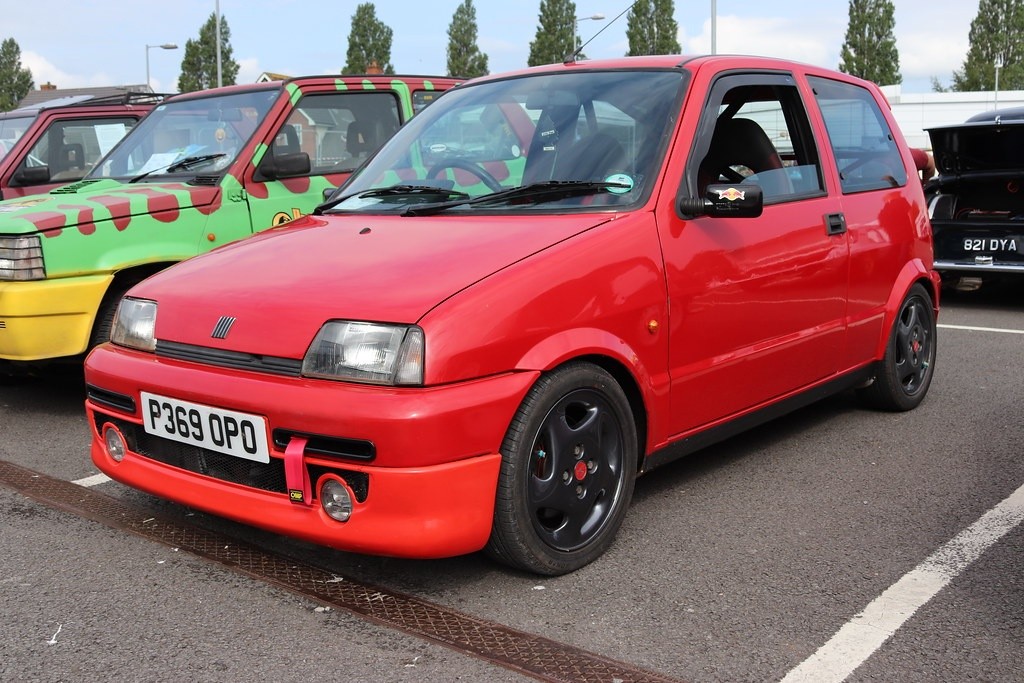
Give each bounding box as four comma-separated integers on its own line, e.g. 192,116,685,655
271,126,302,161
550,133,633,184
688,119,794,200
56,144,86,173
342,119,386,156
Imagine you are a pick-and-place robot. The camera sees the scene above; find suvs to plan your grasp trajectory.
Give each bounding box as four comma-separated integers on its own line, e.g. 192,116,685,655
0,71,541,387
0,94,265,199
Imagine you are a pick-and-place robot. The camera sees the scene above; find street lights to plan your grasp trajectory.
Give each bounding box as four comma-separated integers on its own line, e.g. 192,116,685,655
573,14,605,60
145,43,178,94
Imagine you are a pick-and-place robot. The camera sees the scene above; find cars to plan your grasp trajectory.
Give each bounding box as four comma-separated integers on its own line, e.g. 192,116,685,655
89,54,940,571
915,108,1024,297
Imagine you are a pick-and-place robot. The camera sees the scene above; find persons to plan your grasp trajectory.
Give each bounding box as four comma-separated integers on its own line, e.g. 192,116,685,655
908,147,936,185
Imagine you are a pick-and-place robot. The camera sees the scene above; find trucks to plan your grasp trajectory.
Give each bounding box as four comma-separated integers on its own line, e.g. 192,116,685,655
725,88,1024,168
